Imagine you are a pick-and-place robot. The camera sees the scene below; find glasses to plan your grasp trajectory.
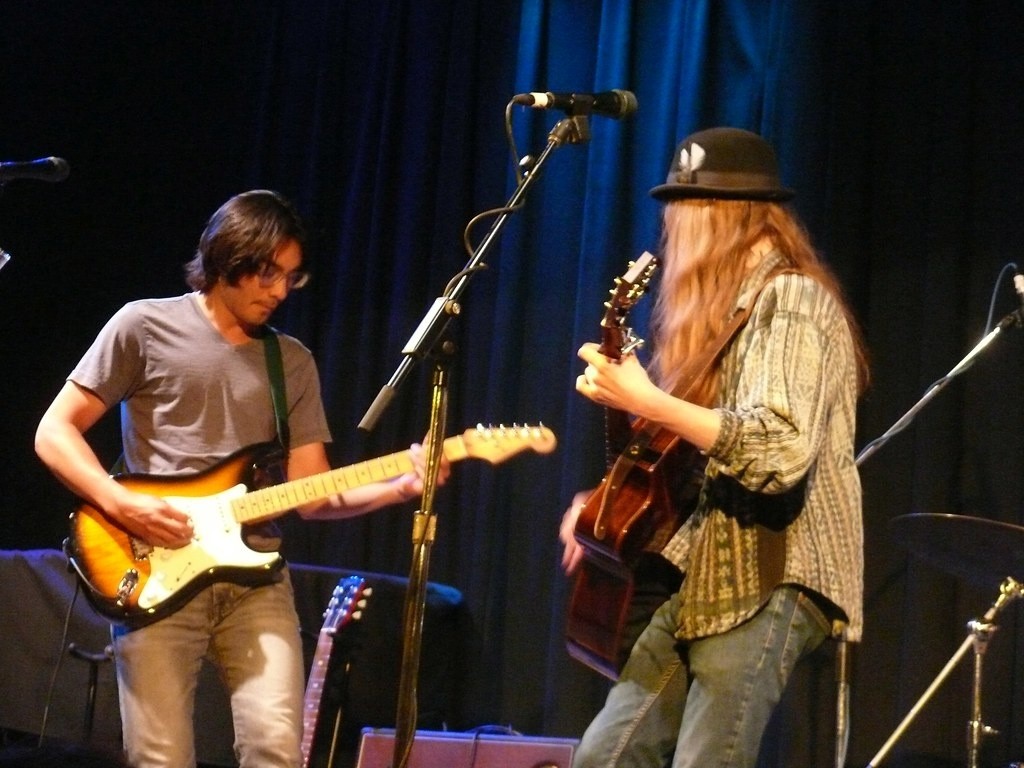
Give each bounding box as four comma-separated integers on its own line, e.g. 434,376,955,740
258,263,312,290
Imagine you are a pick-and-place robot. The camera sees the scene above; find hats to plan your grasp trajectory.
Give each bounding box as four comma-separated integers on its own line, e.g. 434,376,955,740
652,127,798,201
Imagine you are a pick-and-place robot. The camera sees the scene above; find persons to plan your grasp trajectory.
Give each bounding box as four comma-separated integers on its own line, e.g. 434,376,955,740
33,187,450,768
559,125,863,768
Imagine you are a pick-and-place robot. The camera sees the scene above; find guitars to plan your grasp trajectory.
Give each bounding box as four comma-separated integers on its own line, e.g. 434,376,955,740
564,249,694,682
65,419,556,625
301,571,373,768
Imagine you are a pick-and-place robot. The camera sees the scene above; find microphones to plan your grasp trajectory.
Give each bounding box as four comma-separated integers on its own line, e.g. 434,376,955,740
512,88,639,121
1013,271,1024,324
1,155,69,185
